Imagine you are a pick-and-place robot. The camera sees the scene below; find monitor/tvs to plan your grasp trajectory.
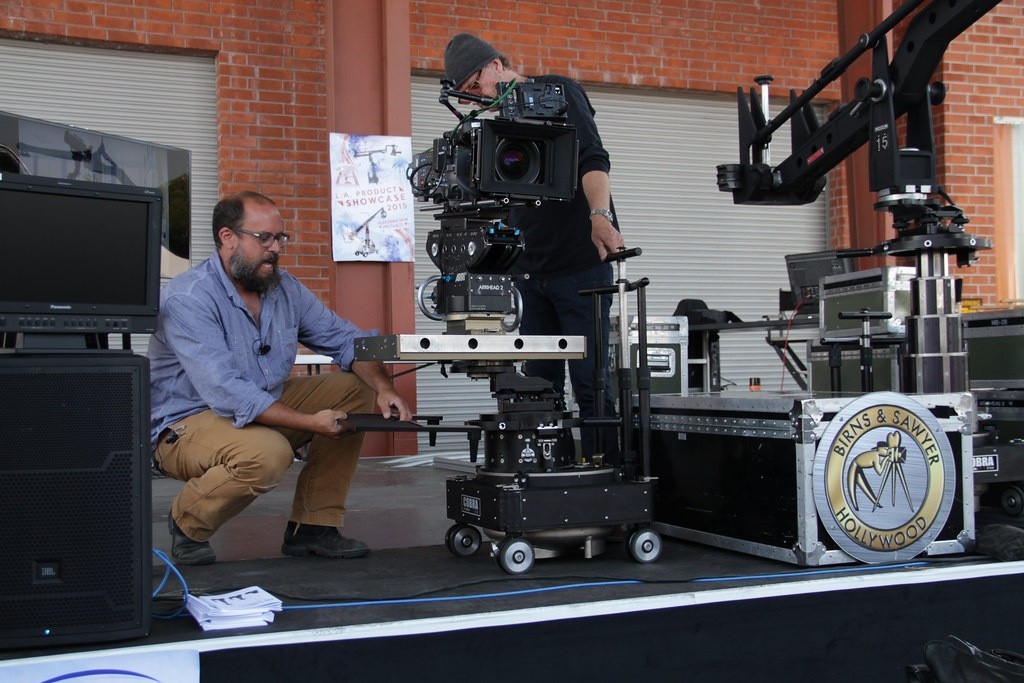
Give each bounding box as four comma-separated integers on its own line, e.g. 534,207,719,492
0,171,163,334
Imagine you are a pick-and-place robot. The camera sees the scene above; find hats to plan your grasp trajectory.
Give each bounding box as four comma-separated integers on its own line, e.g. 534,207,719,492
443,33,502,91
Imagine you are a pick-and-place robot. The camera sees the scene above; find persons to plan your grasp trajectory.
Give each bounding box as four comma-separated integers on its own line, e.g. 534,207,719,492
145,192,412,567
444,34,624,466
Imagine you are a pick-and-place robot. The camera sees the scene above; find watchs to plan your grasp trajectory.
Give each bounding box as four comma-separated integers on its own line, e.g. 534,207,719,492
589,209,614,222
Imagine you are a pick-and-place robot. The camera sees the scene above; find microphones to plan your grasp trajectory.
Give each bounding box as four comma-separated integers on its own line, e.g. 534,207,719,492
261,345,271,356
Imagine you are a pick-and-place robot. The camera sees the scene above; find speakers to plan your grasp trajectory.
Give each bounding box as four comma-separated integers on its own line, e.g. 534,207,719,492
0,348,150,650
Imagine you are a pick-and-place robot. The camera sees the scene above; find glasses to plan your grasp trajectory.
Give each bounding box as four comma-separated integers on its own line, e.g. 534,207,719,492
458,69,482,105
230,227,290,248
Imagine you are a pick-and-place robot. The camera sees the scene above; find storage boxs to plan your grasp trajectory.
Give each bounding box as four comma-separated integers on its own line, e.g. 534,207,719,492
600,264,1024,565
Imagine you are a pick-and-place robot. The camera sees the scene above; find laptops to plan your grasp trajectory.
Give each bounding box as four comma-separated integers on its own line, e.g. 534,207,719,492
785,250,854,315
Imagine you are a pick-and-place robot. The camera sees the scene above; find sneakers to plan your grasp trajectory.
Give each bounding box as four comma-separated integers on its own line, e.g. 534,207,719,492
167,506,217,564
281,517,370,557
